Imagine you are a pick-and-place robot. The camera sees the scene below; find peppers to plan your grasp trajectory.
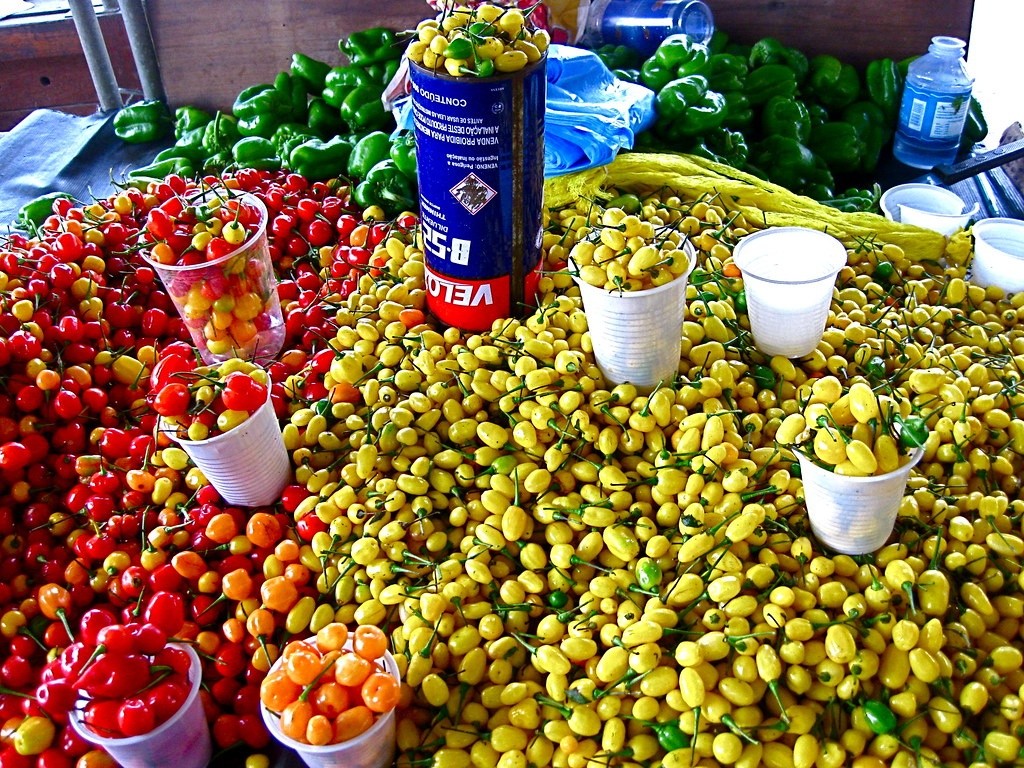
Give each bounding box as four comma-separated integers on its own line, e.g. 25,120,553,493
407,0,552,81
0,165,1024,768
113,28,420,216
588,28,989,219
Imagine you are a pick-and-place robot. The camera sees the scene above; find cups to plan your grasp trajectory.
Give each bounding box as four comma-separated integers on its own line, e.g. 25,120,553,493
138,189,288,367
569,224,696,395
970,218,1024,294
260,631,400,768
155,359,293,508
731,225,849,359
879,180,979,234
409,45,550,335
70,642,212,768
793,430,925,556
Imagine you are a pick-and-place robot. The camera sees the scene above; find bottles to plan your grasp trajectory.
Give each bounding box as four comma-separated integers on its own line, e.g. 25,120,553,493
893,36,974,169
586,0,715,58
